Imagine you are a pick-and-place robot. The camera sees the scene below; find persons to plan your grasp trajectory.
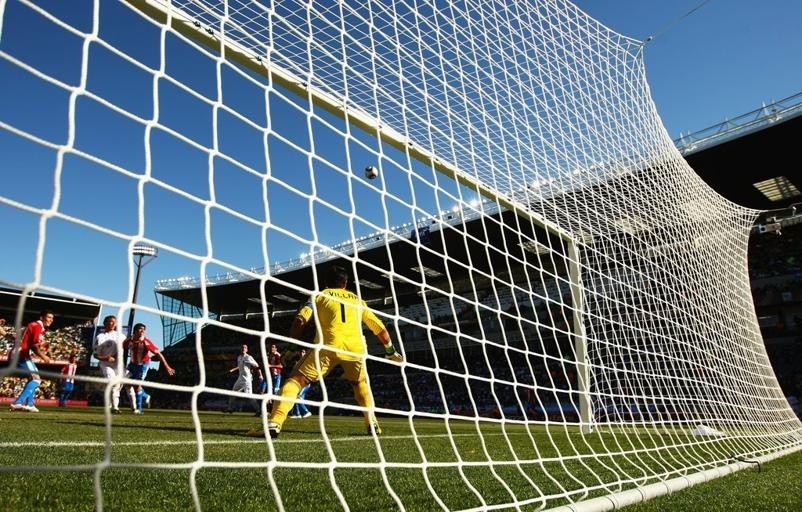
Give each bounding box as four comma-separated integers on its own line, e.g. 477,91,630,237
0,316,95,401
123,322,177,415
259,343,286,418
9,312,55,413
92,314,142,416
243,264,405,439
288,349,314,419
224,344,265,418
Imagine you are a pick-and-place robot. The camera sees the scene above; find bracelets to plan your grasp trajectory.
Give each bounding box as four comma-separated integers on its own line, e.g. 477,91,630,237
286,343,298,350
381,340,396,354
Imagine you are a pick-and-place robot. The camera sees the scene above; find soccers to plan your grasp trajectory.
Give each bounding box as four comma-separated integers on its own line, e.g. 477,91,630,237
365,165,378,179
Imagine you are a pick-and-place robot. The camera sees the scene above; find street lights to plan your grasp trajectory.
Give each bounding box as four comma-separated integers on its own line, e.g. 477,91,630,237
121,243,160,368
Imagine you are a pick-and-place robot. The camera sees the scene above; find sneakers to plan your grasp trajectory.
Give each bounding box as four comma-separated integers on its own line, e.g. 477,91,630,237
111,409,122,415
10,404,23,411
246,422,279,439
221,409,233,413
367,423,382,436
254,411,261,417
24,404,39,412
303,412,312,418
290,415,302,418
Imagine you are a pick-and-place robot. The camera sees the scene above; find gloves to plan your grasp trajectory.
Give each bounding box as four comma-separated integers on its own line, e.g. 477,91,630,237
384,345,403,363
280,349,299,368
